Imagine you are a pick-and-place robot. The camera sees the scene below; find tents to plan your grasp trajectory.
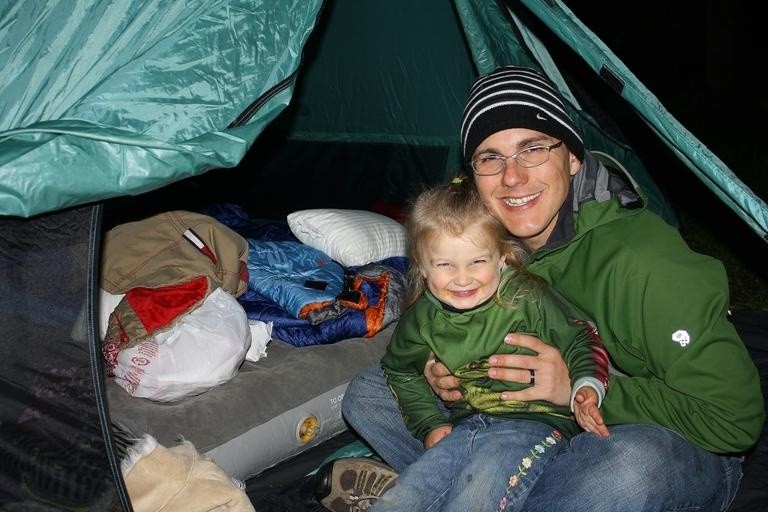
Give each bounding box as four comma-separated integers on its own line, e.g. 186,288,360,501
0,1,766,512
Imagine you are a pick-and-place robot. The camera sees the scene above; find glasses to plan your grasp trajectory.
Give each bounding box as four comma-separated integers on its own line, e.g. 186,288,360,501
468,141,563,176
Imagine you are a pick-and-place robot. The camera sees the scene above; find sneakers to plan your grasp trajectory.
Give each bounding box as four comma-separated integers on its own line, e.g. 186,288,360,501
314,458,399,512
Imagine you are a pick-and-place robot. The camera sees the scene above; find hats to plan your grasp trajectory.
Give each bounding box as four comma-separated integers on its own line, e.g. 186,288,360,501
461,65,585,166
103,277,212,376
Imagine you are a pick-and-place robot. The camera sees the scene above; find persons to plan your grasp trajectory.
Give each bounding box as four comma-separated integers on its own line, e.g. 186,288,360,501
363,175,613,511
342,64,766,511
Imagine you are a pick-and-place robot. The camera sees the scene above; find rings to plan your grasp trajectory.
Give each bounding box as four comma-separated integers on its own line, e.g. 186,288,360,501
527,369,536,384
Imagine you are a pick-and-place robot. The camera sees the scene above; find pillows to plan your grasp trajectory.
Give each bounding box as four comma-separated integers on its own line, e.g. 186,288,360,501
284,207,411,268
